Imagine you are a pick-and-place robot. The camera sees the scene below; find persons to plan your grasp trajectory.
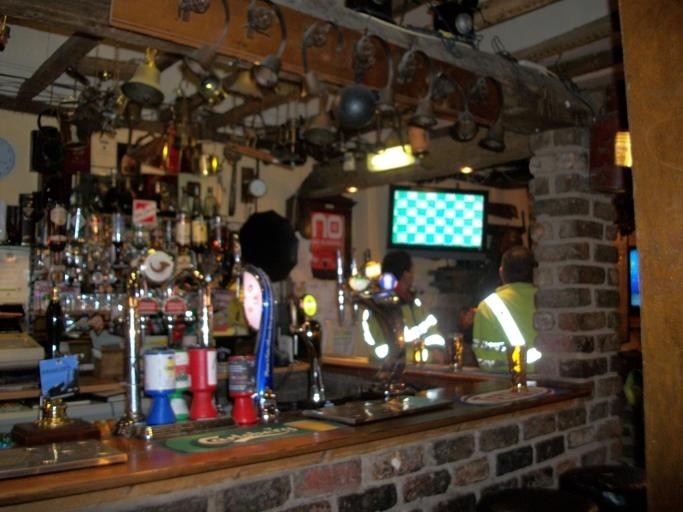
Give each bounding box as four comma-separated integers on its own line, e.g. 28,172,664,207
362,251,443,385
469,246,537,389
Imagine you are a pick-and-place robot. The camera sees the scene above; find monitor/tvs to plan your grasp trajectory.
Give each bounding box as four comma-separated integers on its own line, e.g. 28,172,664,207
627,245,640,315
388,184,490,262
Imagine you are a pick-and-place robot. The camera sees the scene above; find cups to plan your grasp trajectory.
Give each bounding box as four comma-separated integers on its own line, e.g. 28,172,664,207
445,331,465,373
507,345,528,392
413,340,423,367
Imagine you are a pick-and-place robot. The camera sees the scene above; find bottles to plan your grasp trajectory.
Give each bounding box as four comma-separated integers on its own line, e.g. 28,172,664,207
176,191,190,246
205,186,214,215
211,214,227,251
43,286,66,361
189,185,208,250
